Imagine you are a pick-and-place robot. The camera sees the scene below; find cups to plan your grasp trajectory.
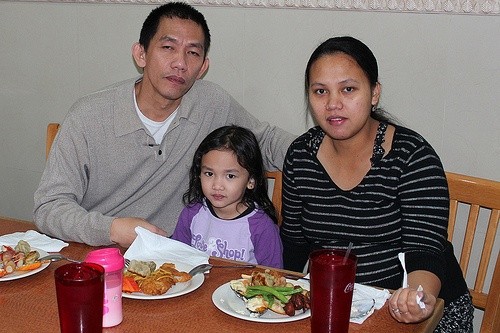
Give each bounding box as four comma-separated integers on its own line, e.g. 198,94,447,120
54,262,105,333
308,249,357,333
83,248,125,328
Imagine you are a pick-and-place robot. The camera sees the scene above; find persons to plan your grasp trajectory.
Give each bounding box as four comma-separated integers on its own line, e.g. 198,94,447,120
278,36,474,333
172,125,285,269
33,2,299,249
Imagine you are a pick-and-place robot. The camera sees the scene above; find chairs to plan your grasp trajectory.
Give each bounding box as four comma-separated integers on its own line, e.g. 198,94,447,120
45,123,500,333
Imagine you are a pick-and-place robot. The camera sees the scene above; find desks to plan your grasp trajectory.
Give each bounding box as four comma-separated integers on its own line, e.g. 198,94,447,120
0,217,445,333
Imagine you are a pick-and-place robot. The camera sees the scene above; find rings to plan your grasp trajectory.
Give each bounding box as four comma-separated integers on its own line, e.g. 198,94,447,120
392,308,399,314
400,311,408,315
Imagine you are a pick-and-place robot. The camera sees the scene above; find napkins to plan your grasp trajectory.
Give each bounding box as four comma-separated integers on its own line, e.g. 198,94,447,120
0,230,69,253
123,225,211,274
294,272,391,325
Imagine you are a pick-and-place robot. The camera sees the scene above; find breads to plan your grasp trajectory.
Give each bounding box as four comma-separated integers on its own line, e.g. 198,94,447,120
127,260,156,276
14,240,40,261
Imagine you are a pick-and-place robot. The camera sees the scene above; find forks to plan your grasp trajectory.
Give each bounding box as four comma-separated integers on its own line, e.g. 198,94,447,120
124,258,130,266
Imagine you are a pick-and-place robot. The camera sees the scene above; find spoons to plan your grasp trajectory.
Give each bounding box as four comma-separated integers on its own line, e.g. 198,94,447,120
189,264,256,277
40,253,81,262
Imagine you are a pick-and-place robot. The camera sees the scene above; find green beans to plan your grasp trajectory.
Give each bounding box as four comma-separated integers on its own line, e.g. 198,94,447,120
245,284,303,308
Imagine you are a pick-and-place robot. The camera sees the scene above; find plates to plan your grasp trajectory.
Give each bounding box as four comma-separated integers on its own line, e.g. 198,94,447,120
121,259,205,299
0,245,51,281
212,279,311,323
